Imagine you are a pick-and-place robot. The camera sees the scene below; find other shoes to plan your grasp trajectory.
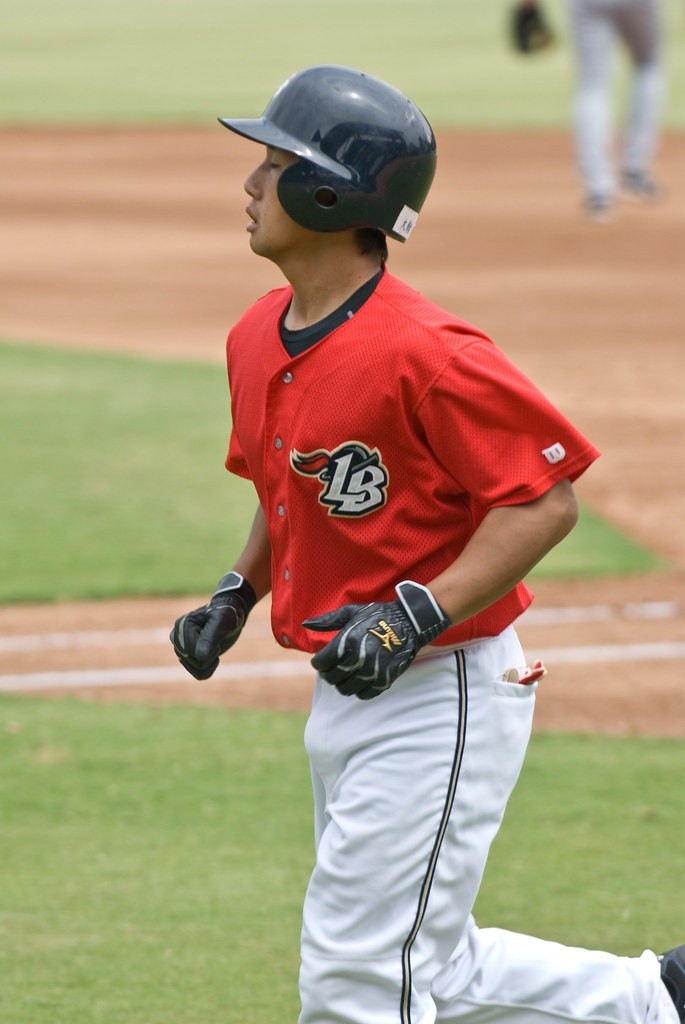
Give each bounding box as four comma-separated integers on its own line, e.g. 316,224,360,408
617,169,654,201
655,944,685,1024
584,193,607,217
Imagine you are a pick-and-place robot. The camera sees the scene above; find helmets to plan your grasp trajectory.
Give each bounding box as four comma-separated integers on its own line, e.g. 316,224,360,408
218,65,438,243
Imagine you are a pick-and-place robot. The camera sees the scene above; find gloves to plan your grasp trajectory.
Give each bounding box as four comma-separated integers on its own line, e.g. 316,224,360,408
503,660,547,685
170,572,258,680
302,580,451,701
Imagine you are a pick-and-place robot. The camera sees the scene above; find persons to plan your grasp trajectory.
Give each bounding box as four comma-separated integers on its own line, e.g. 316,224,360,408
512,0,671,221
168,64,684,1024
